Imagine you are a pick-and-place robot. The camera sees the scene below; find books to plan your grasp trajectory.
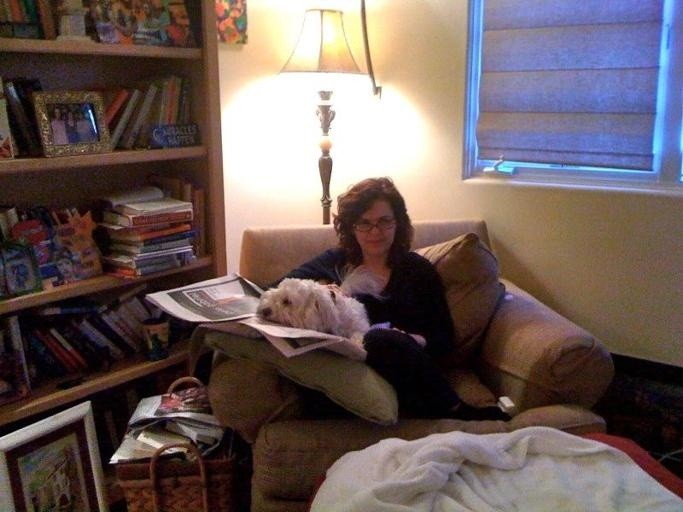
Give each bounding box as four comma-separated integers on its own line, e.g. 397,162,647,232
102,77,194,149
0,74,45,159
0,0,41,39
0,201,79,240
0,284,224,460
97,173,209,279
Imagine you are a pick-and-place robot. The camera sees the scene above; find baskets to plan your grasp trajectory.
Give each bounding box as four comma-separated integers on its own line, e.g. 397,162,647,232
116,377,236,512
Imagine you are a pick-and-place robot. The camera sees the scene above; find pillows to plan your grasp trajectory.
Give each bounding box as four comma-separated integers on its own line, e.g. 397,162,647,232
409,231,513,352
196,325,424,429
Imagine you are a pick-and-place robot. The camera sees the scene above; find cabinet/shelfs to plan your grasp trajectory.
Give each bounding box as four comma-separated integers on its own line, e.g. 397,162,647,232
1,1,231,512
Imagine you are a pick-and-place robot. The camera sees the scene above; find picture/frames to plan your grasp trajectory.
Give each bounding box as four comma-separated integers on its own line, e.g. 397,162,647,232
31,90,114,158
2,400,114,512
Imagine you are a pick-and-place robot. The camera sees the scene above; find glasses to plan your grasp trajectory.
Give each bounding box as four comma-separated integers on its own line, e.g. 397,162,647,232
352,219,396,231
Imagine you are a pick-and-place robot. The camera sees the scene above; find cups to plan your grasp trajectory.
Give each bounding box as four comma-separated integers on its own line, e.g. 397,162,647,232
142,317,170,358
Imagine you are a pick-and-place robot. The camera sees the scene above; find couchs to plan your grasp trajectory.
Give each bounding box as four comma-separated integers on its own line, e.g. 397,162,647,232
206,217,614,511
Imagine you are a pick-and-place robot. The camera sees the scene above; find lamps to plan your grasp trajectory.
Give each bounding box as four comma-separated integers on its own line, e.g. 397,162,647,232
281,3,369,228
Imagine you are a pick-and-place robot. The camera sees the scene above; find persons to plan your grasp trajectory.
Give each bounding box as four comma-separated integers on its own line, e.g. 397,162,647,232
49,247,80,285
105,3,166,46
51,108,93,144
261,177,510,423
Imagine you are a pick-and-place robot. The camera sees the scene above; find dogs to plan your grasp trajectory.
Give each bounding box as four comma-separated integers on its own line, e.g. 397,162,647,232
255,276,371,351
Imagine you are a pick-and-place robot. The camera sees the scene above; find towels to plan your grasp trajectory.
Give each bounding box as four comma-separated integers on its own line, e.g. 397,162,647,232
306,424,682,511
